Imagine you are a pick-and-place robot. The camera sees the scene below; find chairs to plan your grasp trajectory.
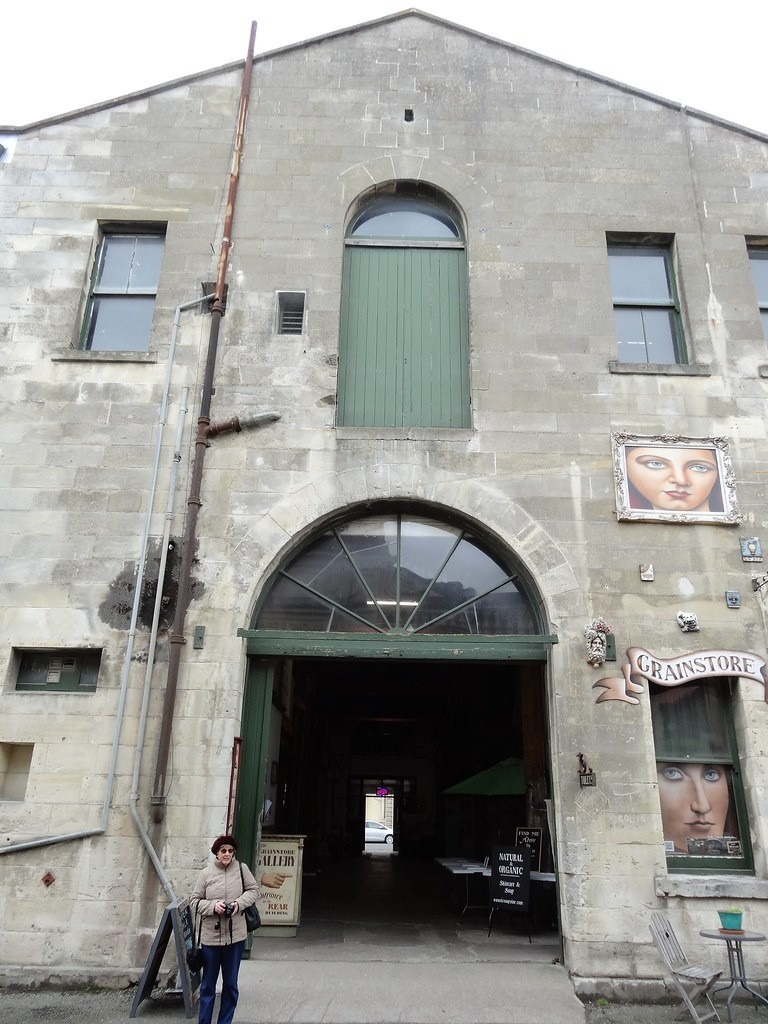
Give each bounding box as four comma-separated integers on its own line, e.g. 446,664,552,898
648,910,724,1024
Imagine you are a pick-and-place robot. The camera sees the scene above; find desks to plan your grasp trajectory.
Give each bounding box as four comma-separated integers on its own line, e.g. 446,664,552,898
436,857,556,926
700,929,768,1022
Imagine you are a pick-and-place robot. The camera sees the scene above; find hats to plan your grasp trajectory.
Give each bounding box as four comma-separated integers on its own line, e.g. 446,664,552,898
211,836,238,855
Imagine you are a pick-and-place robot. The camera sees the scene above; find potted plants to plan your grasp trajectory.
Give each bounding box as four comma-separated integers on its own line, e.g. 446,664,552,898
718,906,742,931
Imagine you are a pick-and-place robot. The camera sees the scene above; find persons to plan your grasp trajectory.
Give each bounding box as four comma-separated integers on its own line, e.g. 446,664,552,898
587,631,606,667
190,836,258,1024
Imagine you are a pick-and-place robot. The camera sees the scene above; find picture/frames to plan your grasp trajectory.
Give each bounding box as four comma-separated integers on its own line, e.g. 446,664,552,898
609,431,742,526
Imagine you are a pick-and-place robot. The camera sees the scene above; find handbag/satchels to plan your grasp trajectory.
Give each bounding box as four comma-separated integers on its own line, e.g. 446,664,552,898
186,948,203,972
239,861,261,932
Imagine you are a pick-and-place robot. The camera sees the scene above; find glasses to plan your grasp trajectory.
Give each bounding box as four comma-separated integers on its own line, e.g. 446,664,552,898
221,849,234,853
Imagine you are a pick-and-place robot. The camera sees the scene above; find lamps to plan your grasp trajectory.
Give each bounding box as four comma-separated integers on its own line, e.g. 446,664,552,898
376,780,388,796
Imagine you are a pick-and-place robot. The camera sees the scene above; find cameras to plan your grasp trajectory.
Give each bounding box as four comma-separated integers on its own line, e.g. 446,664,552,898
221,902,234,918
